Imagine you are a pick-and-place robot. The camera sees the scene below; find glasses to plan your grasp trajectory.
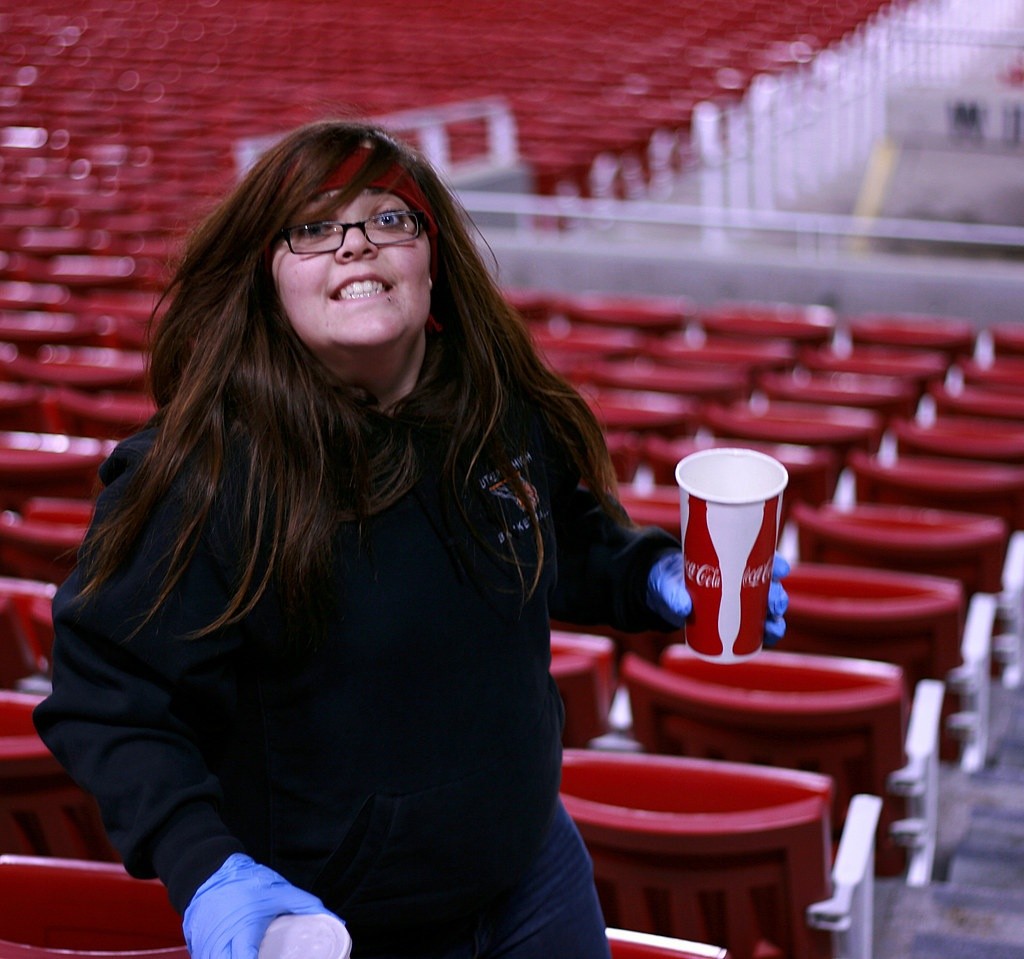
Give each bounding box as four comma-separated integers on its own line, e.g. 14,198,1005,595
278,211,424,254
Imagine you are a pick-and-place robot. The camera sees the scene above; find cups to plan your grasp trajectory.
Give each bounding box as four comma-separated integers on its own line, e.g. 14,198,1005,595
674,449,790,664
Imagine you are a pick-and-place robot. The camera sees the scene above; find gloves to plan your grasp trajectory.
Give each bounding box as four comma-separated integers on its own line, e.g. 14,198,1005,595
650,549,790,642
182,852,346,959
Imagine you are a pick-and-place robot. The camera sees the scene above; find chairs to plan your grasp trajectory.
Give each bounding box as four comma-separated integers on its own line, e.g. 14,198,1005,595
1,0,1024,959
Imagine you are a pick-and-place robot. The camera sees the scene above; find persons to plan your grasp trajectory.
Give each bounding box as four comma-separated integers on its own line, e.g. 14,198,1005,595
30,120,791,959
